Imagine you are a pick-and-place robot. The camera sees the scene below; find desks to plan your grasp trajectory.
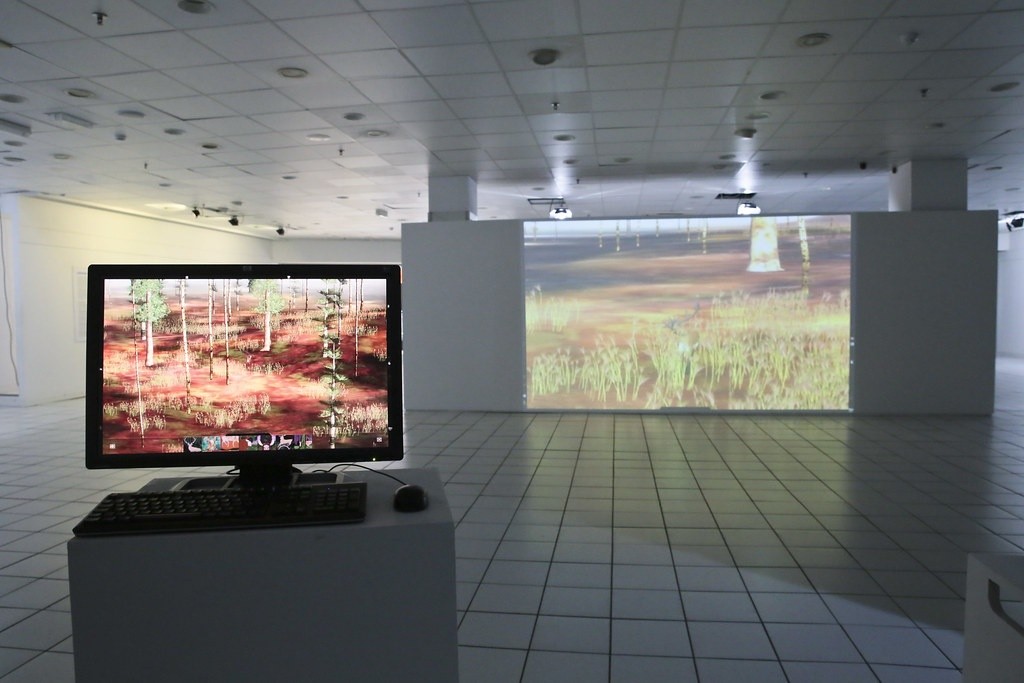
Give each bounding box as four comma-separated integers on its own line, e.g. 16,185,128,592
67,466,459,683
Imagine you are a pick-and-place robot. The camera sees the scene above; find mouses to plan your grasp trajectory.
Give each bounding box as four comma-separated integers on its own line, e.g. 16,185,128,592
392,483,429,513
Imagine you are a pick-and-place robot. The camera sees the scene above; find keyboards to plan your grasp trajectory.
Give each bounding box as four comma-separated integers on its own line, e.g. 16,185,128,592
72,482,367,538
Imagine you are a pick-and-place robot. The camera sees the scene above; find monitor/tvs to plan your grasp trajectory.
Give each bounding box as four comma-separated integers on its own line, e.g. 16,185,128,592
83,263,402,489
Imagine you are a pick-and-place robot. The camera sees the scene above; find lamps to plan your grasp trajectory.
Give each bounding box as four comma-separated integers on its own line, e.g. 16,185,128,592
275,228,284,236
228,217,238,226
1011,217,1024,228
192,208,200,218
548,199,572,221
737,197,761,216
